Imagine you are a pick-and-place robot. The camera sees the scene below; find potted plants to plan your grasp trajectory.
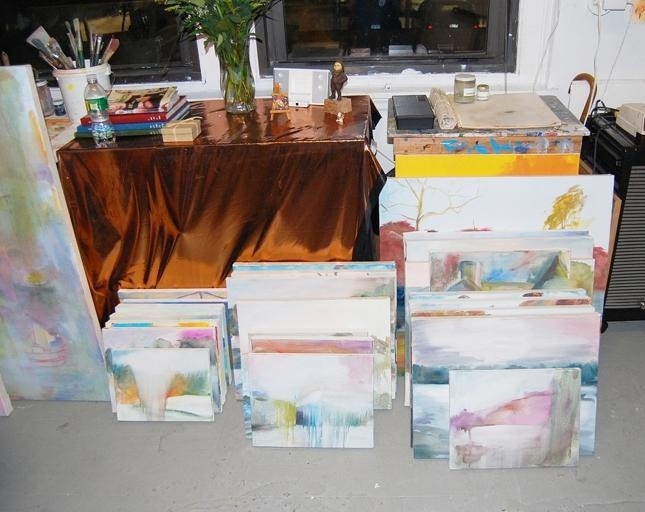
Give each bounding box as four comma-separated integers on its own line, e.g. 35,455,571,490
157,1,283,116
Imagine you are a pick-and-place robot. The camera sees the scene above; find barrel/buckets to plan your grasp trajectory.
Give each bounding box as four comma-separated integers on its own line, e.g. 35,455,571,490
52,59,116,128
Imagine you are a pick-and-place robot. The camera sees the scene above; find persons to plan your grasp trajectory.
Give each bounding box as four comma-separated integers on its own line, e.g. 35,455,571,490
324,58,352,125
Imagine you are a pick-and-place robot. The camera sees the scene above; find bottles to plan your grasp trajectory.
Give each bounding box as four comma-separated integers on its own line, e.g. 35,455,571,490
84,74,116,146
454,74,491,104
35,79,66,116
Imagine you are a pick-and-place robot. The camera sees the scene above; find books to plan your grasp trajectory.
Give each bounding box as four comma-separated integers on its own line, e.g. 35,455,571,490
74,86,191,138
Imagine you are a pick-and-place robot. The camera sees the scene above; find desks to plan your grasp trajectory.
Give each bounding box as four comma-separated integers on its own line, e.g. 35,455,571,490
386,94,593,172
52,96,378,295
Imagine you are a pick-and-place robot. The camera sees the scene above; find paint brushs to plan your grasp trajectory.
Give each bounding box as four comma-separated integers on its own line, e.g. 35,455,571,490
26,16,120,70
